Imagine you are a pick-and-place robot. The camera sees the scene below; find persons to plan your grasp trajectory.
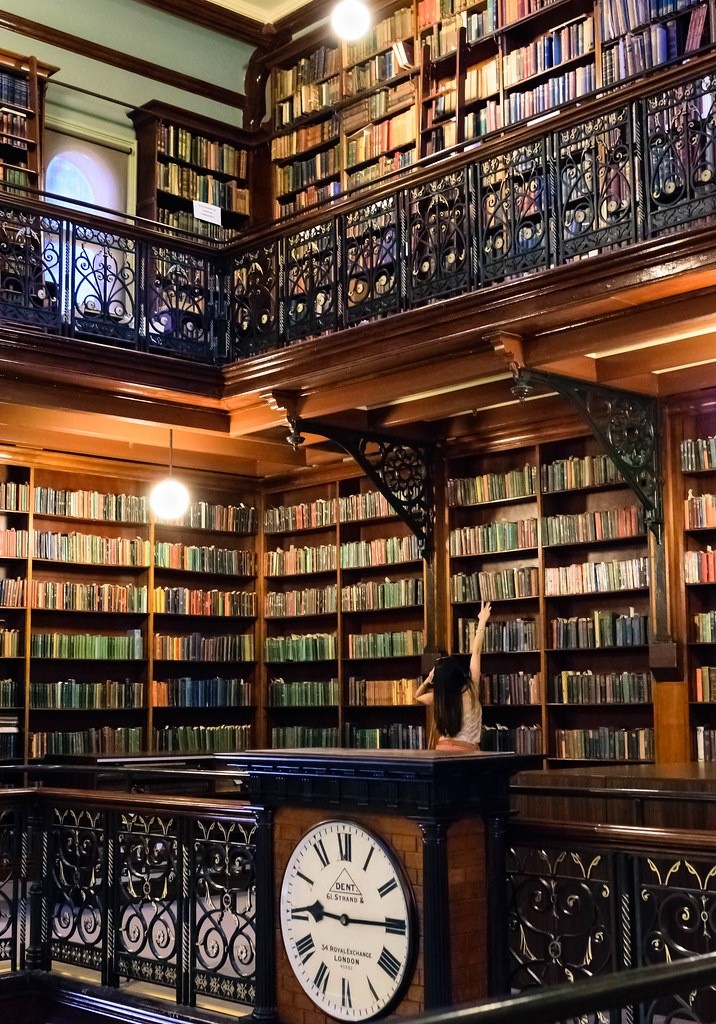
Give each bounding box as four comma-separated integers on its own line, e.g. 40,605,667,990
413,600,491,753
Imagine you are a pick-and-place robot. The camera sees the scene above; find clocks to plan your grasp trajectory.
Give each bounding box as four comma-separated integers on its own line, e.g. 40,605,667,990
279,819,419,1024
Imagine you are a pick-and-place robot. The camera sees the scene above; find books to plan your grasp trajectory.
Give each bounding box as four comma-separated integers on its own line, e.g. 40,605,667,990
0,0,716,342
0,433,716,763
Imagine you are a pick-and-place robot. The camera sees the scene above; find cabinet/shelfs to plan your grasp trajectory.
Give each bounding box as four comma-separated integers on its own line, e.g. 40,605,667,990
0,47,62,333
126,0,716,361
0,387,716,909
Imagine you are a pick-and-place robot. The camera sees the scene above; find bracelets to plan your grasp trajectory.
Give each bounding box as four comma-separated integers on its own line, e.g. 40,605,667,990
423,683,426,686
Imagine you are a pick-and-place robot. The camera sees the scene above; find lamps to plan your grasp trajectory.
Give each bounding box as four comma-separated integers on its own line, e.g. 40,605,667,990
149,429,190,520
